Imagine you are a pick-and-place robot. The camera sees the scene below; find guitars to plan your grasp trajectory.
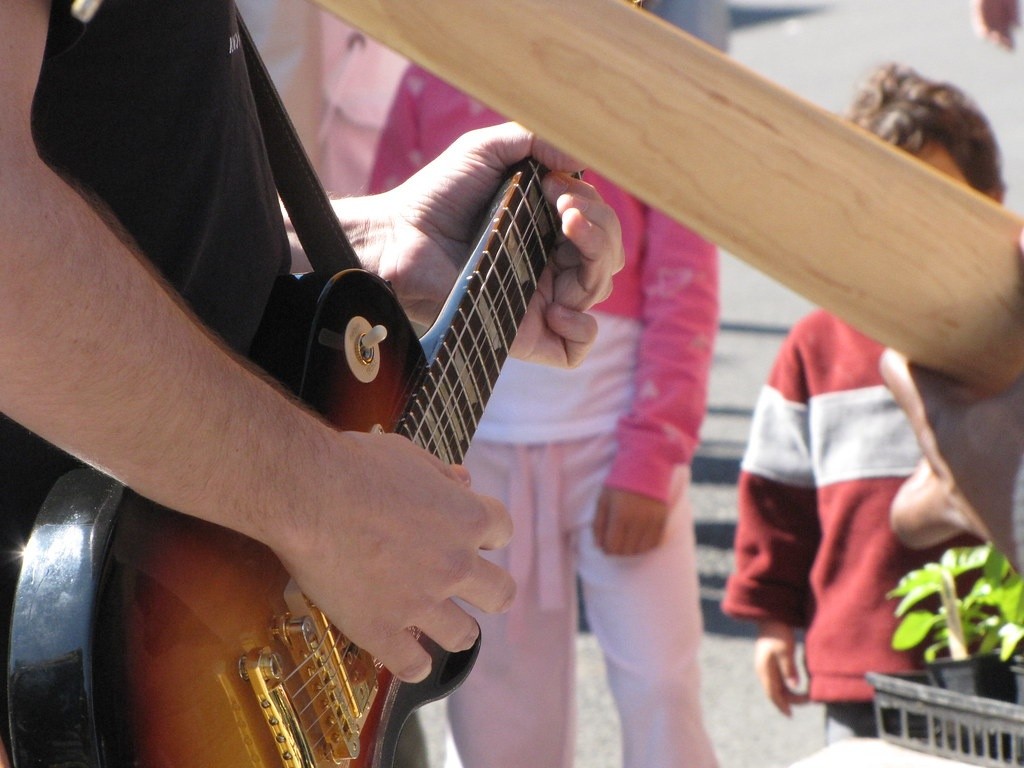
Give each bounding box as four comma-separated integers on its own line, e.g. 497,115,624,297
5,156,596,768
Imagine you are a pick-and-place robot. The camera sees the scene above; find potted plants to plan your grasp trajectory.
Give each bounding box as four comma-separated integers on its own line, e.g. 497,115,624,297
885,540,1023,759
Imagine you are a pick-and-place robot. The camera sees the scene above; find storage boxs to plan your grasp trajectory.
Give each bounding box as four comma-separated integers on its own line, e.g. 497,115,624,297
863,668,1024,768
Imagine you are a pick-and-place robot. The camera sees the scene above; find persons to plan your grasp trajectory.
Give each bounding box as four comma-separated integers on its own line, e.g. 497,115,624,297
0,0,714,768
719,63,1019,747
